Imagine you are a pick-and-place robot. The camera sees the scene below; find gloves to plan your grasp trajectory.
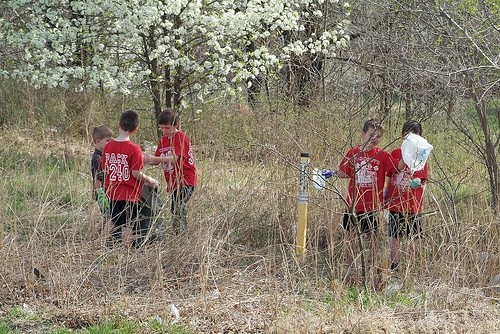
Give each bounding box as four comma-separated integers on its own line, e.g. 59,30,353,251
410,178,421,187
383,189,388,200
321,170,335,178
97,188,109,211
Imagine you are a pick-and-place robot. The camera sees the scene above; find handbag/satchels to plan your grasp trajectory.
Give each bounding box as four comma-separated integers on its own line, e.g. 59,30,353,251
401,132,433,177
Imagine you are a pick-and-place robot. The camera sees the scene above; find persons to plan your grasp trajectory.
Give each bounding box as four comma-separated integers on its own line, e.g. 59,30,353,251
99,110,160,250
322,118,408,267
382,120,430,271
90,123,121,247
143,108,198,241
